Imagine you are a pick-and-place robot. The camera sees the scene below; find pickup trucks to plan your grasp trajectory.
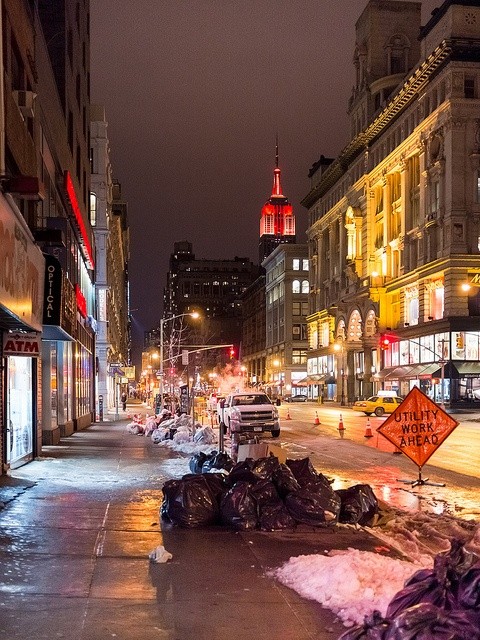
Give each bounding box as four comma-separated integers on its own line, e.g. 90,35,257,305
217,392,281,441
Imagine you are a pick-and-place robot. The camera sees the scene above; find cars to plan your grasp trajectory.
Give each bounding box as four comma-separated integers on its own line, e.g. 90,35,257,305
353,390,405,417
212,396,225,414
284,395,308,402
207,393,218,408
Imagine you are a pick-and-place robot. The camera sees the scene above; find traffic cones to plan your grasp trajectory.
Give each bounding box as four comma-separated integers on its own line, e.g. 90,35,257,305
338,414,345,430
392,443,402,454
365,419,373,437
314,412,321,425
286,408,291,420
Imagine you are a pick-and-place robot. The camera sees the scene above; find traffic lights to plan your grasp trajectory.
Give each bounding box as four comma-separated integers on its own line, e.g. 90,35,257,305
230,346,234,359
382,335,388,351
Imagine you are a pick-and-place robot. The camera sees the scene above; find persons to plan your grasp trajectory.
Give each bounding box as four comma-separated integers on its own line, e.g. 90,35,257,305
120,392,129,411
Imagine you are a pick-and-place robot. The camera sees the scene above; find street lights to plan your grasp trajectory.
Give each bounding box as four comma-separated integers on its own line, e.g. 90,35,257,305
240,367,249,390
273,359,284,398
150,353,158,402
333,339,345,407
160,312,199,410
